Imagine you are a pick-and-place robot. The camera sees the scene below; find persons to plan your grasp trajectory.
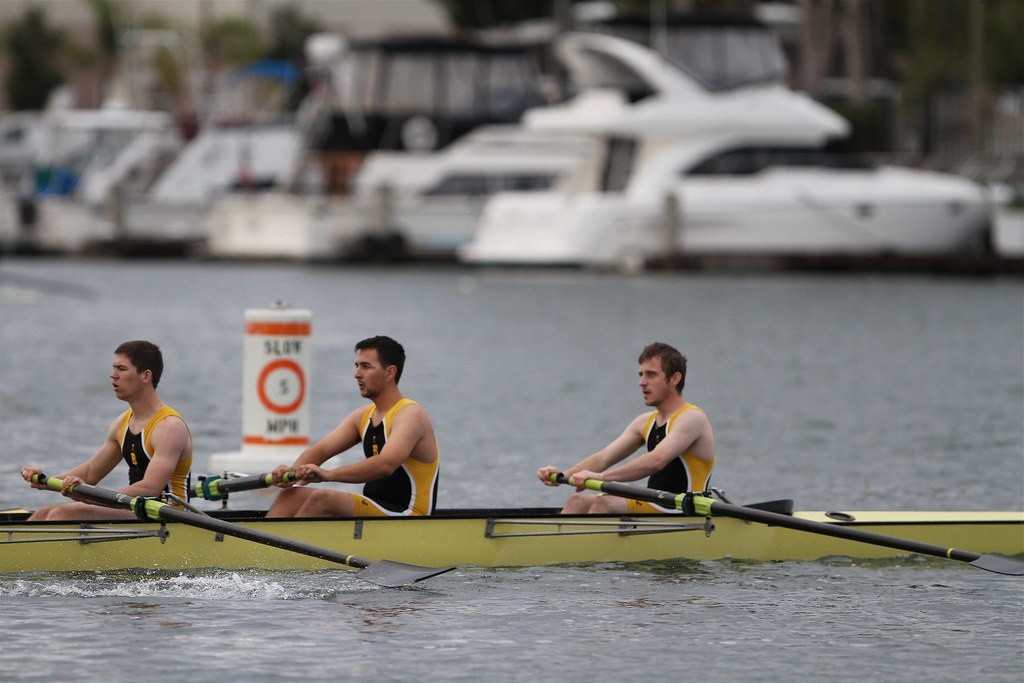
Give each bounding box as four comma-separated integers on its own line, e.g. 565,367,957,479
537,343,716,514
20,341,192,521
267,336,439,517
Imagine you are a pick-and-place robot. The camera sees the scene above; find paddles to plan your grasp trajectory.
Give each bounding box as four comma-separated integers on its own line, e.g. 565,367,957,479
550,472,1024,576
33,469,456,588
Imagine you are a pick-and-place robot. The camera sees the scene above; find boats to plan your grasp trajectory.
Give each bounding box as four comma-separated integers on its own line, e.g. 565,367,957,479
0,25,1024,282
0,496,1024,574
